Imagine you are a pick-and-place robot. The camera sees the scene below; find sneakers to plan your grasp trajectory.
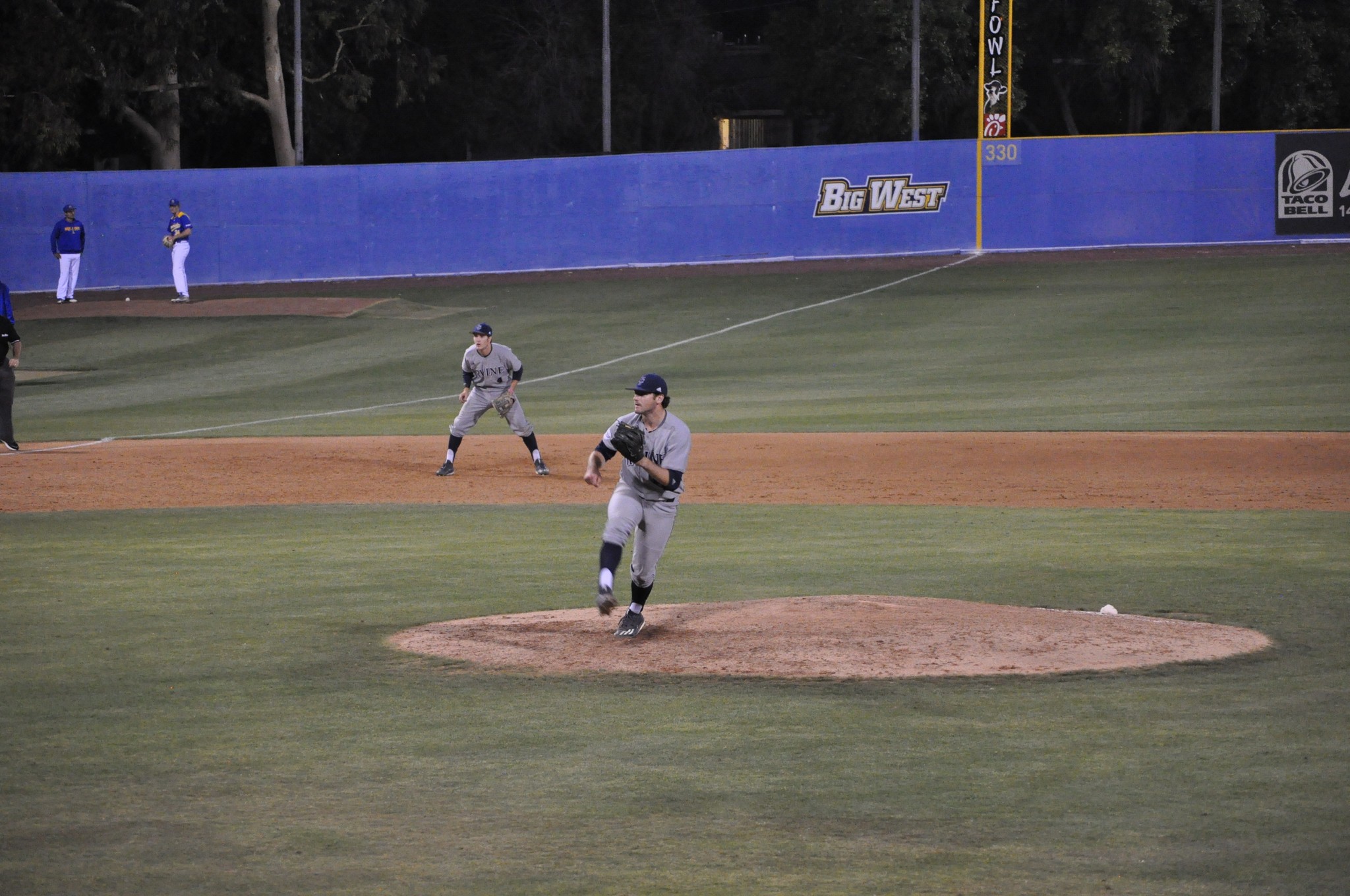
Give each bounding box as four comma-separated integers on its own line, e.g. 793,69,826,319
436,460,455,477
596,585,616,616
0,434,19,452
613,608,644,638
534,458,549,475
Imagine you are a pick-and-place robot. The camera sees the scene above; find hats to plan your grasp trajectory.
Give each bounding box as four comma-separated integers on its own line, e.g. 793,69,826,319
168,199,179,206
63,204,76,212
469,323,492,336
625,373,668,394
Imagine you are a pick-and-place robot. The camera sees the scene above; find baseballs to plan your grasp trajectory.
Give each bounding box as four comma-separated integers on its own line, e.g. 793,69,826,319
125,297,130,302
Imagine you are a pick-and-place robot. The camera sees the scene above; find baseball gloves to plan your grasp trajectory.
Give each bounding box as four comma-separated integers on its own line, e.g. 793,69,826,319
162,236,175,248
491,389,516,419
609,418,644,463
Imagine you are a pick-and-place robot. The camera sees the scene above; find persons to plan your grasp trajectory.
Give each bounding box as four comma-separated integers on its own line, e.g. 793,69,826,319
51,204,85,304
435,324,549,477
583,375,691,639
0,281,23,452
161,198,193,304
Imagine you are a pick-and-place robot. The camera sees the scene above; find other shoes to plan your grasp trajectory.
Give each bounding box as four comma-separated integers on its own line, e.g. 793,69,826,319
57,298,65,304
171,292,190,303
65,296,77,303
